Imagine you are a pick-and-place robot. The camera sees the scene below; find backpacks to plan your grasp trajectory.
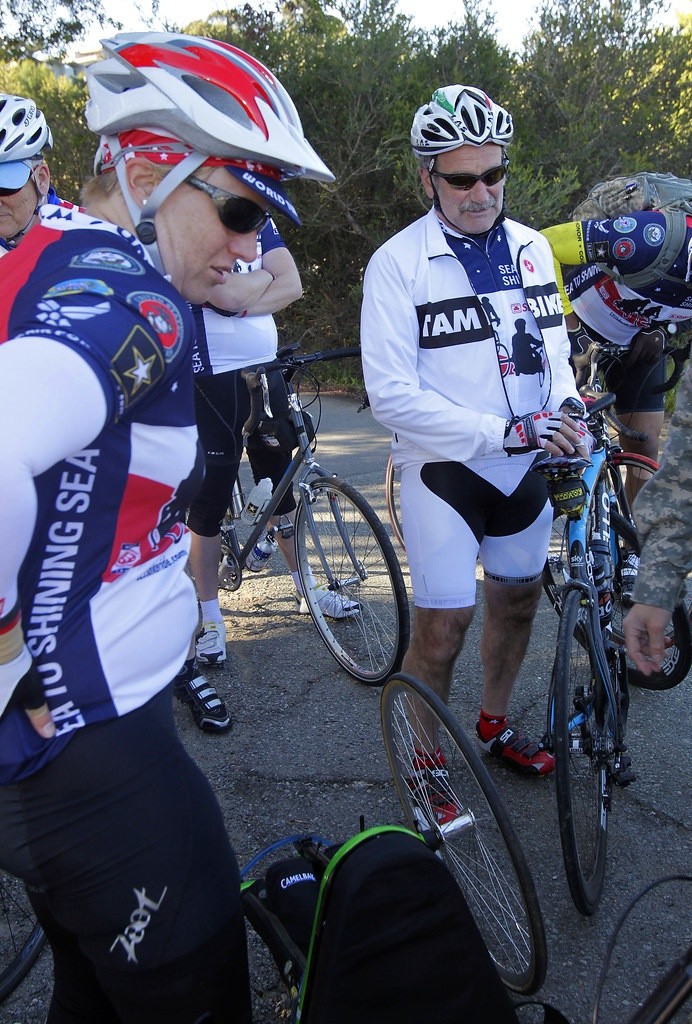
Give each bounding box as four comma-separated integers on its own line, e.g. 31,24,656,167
241,822,514,1024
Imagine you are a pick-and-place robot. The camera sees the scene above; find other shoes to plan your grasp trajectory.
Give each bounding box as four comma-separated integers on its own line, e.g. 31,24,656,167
622,552,641,583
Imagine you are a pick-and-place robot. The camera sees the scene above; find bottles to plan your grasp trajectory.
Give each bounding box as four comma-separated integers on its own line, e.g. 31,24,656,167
593,563,612,625
245,526,276,573
589,483,611,554
240,477,273,525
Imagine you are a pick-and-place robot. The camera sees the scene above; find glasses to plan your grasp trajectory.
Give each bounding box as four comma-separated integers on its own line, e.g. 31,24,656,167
426,157,509,191
0,164,42,197
106,165,272,234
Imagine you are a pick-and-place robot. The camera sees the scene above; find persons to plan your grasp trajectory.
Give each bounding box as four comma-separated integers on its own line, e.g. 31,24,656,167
357,86,589,834
625,362,692,677
188,213,363,664
538,210,692,590
0,94,85,256
0,33,333,1023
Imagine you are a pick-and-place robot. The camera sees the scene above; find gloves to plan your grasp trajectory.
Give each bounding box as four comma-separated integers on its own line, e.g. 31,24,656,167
627,325,667,367
568,323,597,353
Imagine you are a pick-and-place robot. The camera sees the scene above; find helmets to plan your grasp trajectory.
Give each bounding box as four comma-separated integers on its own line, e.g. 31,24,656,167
410,85,513,155
0,94,53,162
86,31,336,184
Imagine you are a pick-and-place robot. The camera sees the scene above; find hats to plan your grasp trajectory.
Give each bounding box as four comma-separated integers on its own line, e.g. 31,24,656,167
231,165,304,229
0,157,32,189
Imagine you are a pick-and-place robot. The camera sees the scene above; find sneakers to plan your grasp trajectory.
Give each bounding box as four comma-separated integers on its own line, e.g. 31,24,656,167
195,621,227,662
409,770,475,835
294,582,362,619
172,670,232,734
474,722,553,774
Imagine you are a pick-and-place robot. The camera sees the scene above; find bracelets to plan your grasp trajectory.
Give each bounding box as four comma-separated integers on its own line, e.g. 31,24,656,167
240,310,247,318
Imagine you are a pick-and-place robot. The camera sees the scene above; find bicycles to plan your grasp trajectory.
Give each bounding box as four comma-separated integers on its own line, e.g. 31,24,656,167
377,669,549,1024
357,391,408,550
494,341,692,917
186,340,411,688
